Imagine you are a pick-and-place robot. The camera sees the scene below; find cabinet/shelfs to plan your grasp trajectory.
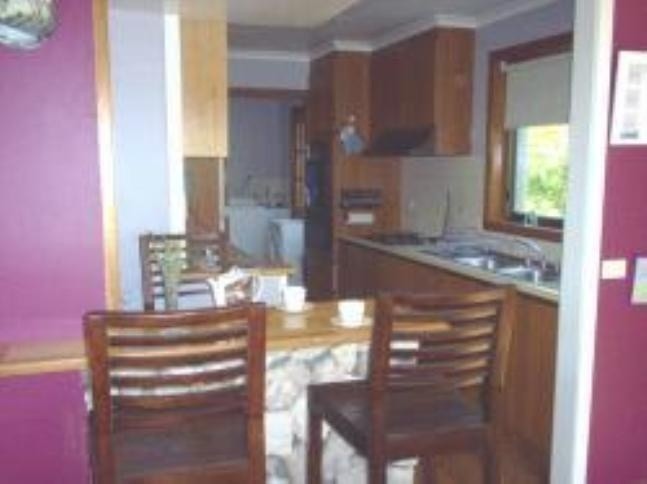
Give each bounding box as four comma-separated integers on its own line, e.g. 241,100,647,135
305,50,401,299
370,28,474,156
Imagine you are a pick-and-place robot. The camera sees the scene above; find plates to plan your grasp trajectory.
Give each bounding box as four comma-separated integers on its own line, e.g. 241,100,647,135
275,305,314,312
328,317,374,329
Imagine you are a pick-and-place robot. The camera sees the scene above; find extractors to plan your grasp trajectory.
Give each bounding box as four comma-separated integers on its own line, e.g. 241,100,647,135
363,124,436,158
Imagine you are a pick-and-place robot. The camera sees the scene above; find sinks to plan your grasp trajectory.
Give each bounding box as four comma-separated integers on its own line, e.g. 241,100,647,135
499,262,561,284
454,252,530,272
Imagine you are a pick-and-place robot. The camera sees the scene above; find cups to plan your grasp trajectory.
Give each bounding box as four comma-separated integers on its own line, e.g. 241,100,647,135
339,300,363,324
282,287,307,307
284,314,306,331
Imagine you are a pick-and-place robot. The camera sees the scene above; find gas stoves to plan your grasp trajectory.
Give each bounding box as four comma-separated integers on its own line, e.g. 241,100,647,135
360,228,437,246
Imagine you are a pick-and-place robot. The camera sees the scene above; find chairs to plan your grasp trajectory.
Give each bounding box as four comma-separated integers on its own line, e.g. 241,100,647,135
84,302,267,484
140,231,232,311
306,286,516,483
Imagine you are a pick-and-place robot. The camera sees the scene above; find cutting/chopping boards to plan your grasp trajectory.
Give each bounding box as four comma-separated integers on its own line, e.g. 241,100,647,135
405,183,448,232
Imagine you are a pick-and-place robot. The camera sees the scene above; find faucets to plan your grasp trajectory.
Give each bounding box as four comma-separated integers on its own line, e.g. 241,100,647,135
502,233,547,273
